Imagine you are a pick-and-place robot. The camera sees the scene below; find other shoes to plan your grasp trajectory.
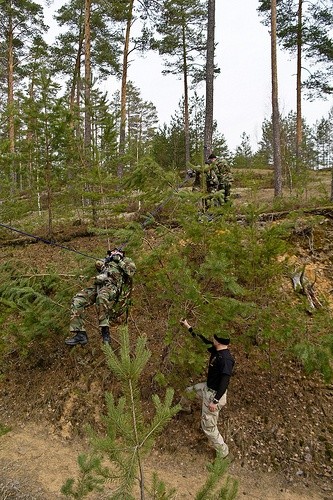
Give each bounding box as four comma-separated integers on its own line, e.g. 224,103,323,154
179,408,191,414
224,451,234,464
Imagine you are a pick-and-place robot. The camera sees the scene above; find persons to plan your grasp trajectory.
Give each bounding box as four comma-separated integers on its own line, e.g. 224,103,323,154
64,246,137,345
186,154,233,215
173,316,235,465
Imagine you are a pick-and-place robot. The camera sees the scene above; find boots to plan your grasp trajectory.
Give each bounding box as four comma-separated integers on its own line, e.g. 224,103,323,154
101,327,111,344
65,332,87,345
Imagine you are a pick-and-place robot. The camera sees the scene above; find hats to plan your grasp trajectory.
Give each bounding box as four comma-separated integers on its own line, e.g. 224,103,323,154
213,333,230,345
